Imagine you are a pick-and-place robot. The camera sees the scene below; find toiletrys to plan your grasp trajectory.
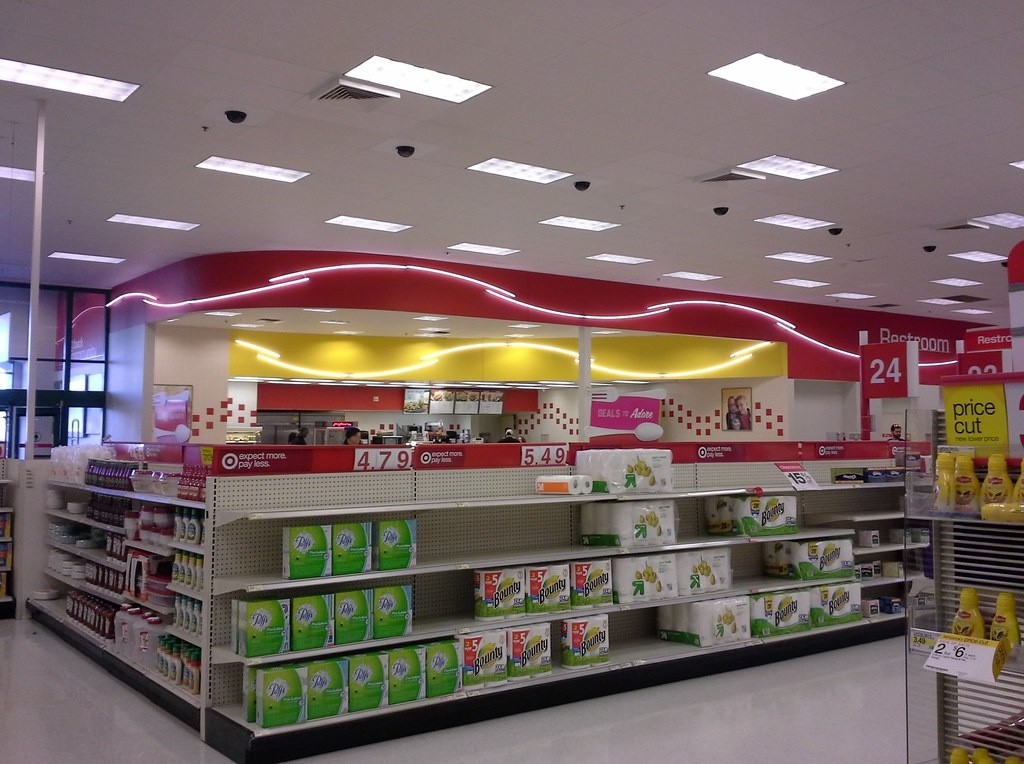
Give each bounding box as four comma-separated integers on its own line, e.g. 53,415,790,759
155,505,206,695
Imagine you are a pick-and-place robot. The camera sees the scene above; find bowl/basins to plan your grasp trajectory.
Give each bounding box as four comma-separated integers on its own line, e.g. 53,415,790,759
145,574,174,606
67,503,86,513
47,489,65,509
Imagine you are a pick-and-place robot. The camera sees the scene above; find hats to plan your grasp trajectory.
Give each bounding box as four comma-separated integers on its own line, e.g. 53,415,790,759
506,429,513,435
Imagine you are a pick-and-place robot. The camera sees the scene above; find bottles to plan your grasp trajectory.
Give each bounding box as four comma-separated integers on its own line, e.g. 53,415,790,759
87,492,132,528
934,453,1024,523
106,532,127,566
951,749,1022,764
114,604,165,670
952,588,1019,646
178,464,212,502
65,587,119,643
124,505,173,541
86,561,124,597
85,459,148,491
129,470,181,496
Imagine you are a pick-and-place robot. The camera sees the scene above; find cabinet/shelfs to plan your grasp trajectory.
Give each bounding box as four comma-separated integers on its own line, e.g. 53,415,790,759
199,336,1024,764
21,443,224,731
0,441,16,618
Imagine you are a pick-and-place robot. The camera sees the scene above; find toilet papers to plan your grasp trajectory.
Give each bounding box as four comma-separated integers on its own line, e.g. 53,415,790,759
579,500,676,547
703,494,797,536
535,448,673,496
452,539,866,692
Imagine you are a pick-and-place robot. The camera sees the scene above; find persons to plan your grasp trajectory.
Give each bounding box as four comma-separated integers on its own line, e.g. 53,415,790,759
293,427,308,444
343,427,361,445
287,432,297,445
497,428,521,443
736,394,751,429
726,396,740,429
887,424,906,441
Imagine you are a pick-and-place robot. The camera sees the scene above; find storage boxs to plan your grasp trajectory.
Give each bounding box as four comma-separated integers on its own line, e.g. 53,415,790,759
125,546,175,608
1,513,12,597
130,470,180,495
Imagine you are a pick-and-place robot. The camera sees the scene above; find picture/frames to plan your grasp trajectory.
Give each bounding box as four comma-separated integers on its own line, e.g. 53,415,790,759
720,387,753,432
